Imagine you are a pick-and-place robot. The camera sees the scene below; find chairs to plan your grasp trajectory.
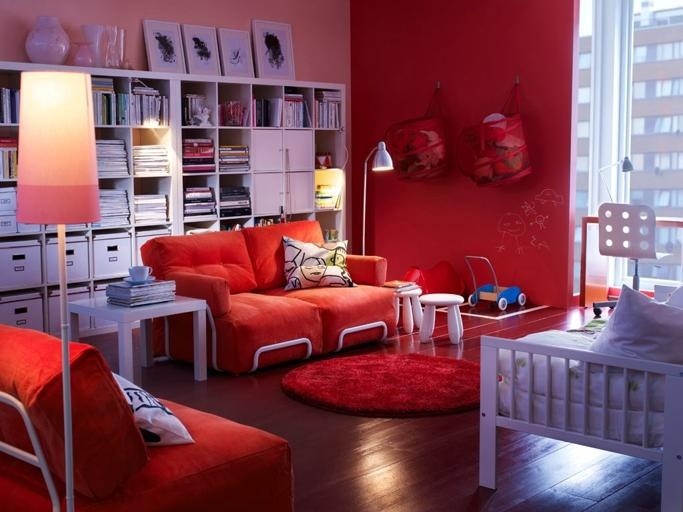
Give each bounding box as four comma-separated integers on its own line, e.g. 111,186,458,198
591,202,674,317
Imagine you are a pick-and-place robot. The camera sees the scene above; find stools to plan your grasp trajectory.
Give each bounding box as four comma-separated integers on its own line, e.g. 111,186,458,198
379,281,424,334
415,293,468,346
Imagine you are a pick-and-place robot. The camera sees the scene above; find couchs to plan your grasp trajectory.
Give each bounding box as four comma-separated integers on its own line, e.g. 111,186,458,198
140,218,401,377
0,319,295,511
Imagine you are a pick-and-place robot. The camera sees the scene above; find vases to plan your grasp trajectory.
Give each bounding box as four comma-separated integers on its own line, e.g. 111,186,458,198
23,13,128,67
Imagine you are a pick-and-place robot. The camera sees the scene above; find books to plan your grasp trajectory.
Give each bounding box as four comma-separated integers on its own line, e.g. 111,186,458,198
218,100,251,230
106,280,178,308
2,85,170,234
182,91,218,235
252,90,341,129
383,278,422,295
254,216,297,227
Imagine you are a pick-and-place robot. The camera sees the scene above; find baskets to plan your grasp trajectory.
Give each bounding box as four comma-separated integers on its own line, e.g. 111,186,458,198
462,112,534,188
390,115,449,182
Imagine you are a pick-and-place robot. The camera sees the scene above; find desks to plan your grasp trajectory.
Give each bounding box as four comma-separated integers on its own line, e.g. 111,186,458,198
579,215,683,307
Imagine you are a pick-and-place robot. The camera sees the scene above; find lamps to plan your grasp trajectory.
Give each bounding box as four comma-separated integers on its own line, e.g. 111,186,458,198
359,139,395,256
14,68,103,511
597,155,634,204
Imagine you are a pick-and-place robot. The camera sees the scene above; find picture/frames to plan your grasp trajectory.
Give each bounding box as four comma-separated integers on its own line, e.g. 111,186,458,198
141,17,299,81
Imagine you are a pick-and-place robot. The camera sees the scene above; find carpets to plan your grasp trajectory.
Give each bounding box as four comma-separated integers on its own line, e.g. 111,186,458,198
280,351,502,420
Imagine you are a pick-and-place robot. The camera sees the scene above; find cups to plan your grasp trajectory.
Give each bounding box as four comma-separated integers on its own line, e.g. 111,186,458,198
128,266,152,281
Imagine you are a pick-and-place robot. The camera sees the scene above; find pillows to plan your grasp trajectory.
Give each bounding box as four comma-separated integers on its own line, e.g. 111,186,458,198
111,371,196,448
587,282,683,367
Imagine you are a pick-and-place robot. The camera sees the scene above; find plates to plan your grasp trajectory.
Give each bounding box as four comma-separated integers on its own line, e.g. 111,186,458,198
123,276,155,284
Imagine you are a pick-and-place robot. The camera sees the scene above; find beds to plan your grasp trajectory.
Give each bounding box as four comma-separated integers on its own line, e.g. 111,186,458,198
476,284,683,511
